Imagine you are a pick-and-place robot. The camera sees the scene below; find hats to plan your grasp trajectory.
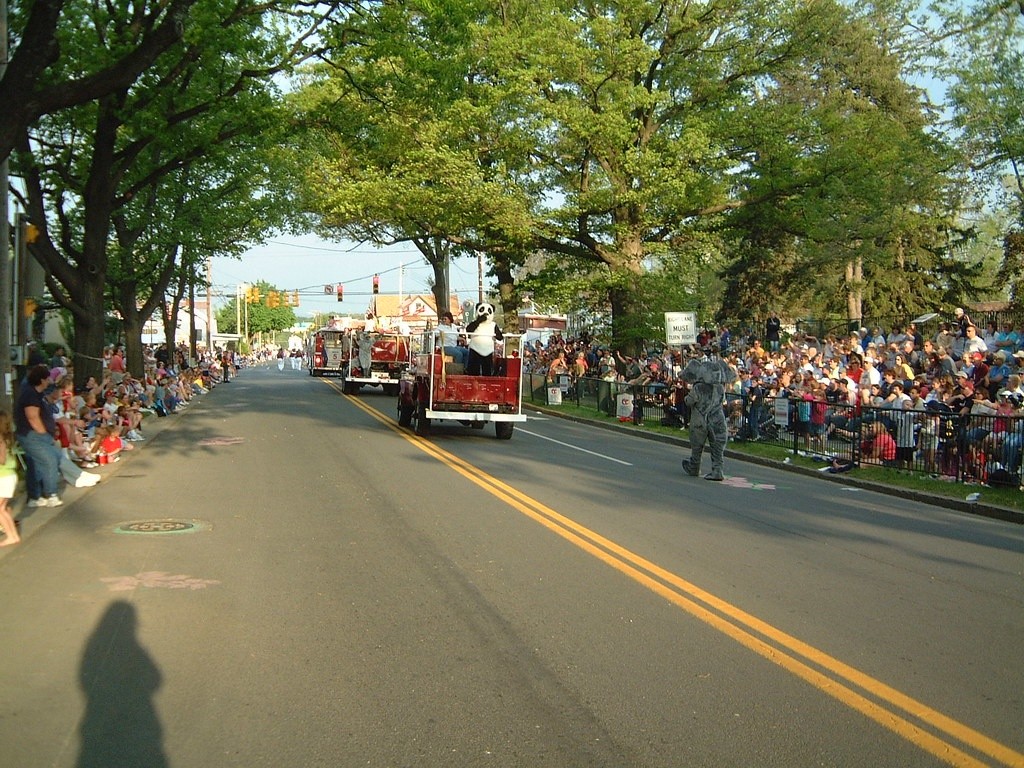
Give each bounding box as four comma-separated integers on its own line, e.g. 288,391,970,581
992,352,1006,361
954,371,968,379
104,390,117,397
959,382,973,390
1012,350,1024,358
970,353,982,360
817,378,829,385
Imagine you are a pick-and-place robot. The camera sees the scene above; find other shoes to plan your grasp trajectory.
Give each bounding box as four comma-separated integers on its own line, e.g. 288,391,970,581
131,434,144,441
48,497,62,507
75,472,100,486
28,497,47,506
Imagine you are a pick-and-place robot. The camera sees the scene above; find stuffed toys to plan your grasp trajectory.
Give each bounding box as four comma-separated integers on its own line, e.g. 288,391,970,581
352,330,382,378
466,302,503,377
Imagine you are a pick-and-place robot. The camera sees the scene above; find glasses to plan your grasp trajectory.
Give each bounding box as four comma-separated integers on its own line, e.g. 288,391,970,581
975,393,981,395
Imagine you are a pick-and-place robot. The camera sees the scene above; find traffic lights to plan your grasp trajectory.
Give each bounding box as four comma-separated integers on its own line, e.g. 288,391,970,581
337,285,343,301
373,276,379,295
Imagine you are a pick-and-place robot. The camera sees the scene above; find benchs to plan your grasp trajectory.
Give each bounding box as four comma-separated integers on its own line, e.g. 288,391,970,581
428,352,454,375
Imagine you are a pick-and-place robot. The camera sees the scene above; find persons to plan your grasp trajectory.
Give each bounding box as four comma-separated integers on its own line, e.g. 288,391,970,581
522,308,1023,489
434,311,469,372
0,340,271,545
277,348,305,370
364,313,378,333
